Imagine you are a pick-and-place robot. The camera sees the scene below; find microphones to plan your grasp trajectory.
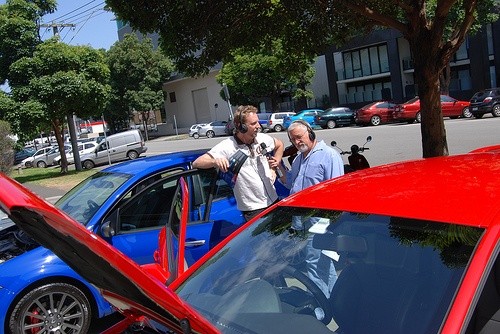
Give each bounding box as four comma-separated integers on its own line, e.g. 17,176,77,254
260,142,284,178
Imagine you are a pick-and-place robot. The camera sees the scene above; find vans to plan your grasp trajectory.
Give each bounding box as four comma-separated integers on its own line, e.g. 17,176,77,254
268,112,296,132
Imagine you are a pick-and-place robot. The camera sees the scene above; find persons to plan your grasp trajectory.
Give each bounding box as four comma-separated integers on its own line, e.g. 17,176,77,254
193,106,286,223
267,120,340,318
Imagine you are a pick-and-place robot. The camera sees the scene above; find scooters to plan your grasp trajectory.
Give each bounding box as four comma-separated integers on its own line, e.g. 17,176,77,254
281,136,374,174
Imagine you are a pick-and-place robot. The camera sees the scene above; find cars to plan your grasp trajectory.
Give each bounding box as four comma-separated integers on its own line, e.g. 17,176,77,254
10,126,148,170
188,111,269,138
469,88,500,118
1,145,280,334
392,94,472,123
355,101,397,127
283,109,324,131
0,143,499,334
314,107,354,129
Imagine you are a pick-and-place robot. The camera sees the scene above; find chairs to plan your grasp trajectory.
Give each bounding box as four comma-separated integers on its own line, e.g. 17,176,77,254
332,234,499,334
115,175,181,227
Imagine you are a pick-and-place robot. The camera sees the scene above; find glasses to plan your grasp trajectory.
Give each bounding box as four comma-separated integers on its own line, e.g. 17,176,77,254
289,129,307,142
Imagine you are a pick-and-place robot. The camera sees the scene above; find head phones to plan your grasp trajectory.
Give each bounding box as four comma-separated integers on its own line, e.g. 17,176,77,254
237,107,248,133
287,121,316,141
